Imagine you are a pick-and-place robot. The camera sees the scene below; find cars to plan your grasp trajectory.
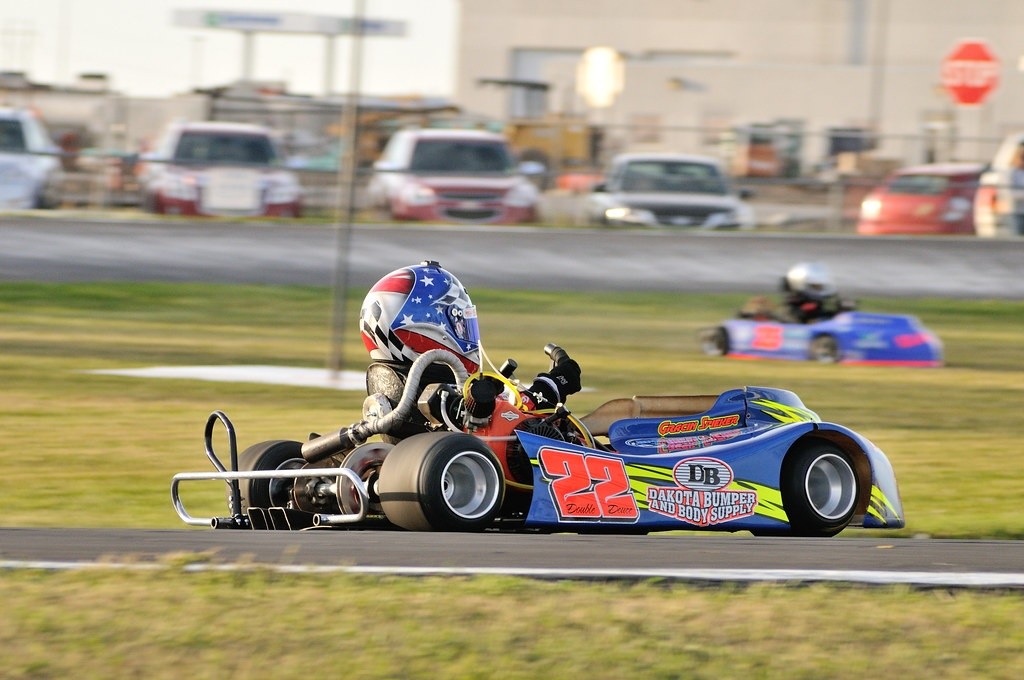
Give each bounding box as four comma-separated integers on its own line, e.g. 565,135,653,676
856,160,982,238
1,107,63,213
369,125,546,226
976,136,1024,241
584,150,750,232
136,118,306,220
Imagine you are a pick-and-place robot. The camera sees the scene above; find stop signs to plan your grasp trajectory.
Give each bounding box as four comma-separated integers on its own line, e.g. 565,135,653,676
945,41,998,103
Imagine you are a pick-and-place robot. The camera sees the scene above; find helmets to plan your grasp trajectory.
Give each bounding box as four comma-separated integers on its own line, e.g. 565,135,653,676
782,262,841,324
359,260,481,375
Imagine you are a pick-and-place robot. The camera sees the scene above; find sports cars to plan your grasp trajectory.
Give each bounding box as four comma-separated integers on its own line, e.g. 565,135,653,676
704,296,945,372
160,339,910,539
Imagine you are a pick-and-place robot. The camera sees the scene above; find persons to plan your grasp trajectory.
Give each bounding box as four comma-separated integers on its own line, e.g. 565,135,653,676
359,261,583,445
747,265,856,322
